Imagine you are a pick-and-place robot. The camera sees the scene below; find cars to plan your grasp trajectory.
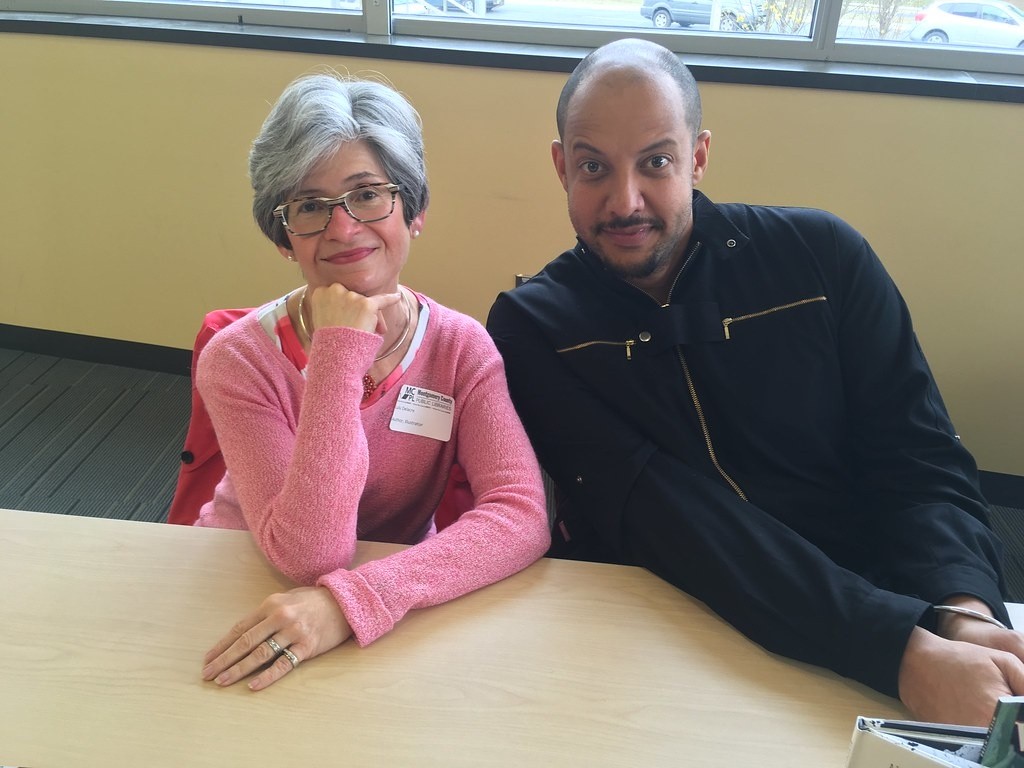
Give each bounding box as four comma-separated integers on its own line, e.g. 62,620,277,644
426,0,504,12
339,0,443,14
910,0,1024,48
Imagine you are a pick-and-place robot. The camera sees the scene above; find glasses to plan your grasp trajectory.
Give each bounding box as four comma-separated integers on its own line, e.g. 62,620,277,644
273,183,400,236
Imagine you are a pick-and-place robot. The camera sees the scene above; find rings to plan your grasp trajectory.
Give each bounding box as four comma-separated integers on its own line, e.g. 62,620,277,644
281,648,300,668
266,637,283,656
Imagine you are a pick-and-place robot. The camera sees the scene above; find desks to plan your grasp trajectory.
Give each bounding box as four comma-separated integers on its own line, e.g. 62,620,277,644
1,501,1024,768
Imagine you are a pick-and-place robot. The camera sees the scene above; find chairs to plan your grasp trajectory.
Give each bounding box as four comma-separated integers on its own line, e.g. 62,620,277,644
167,306,255,526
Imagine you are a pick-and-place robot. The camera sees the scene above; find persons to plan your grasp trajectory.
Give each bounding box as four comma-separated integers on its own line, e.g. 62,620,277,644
193,63,552,692
485,37,1024,727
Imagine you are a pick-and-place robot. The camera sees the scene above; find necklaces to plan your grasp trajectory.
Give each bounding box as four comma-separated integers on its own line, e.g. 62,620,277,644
298,285,413,402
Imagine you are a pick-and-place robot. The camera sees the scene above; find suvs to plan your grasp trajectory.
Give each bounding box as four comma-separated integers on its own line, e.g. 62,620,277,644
641,0,768,32
